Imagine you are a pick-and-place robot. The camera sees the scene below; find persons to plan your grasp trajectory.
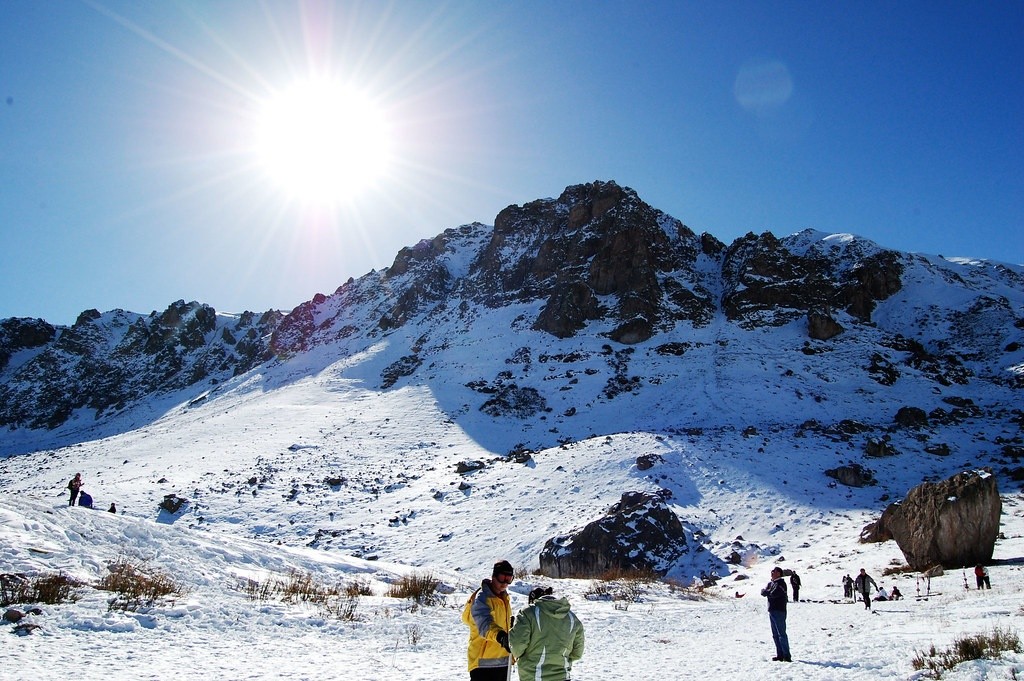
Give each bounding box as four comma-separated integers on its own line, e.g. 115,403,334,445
506,586,586,681
461,560,516,681
66,473,85,507
760,566,792,662
789,570,801,602
982,565,992,589
842,568,903,610
108,503,116,514
78,490,93,510
975,562,986,590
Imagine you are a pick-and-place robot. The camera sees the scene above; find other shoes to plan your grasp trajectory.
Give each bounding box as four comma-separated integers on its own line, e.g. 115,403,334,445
773,657,782,661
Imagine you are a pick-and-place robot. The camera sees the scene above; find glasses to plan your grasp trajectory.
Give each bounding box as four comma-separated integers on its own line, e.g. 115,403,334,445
495,573,515,584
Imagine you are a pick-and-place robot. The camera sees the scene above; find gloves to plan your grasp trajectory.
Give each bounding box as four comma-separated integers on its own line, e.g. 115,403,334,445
496,630,511,653
